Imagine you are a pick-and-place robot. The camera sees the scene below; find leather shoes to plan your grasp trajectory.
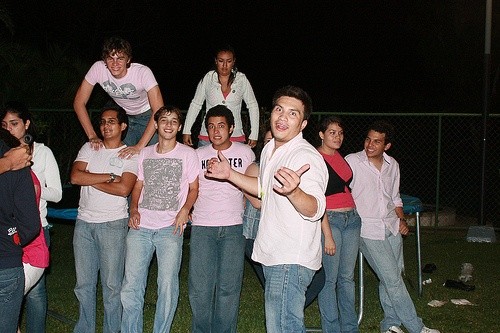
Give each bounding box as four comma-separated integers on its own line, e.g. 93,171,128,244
424,263,436,274
445,279,475,291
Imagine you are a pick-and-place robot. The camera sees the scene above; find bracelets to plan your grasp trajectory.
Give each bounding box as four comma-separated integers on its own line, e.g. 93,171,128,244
400,217,407,224
110,173,116,183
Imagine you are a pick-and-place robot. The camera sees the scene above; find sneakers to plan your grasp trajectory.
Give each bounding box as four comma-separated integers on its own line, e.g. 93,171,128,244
419,325,440,333
381,325,404,333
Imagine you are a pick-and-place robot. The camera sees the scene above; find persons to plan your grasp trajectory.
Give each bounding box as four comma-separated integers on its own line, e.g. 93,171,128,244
344,121,443,333
316,116,362,333
70,36,330,333
0,104,63,333
